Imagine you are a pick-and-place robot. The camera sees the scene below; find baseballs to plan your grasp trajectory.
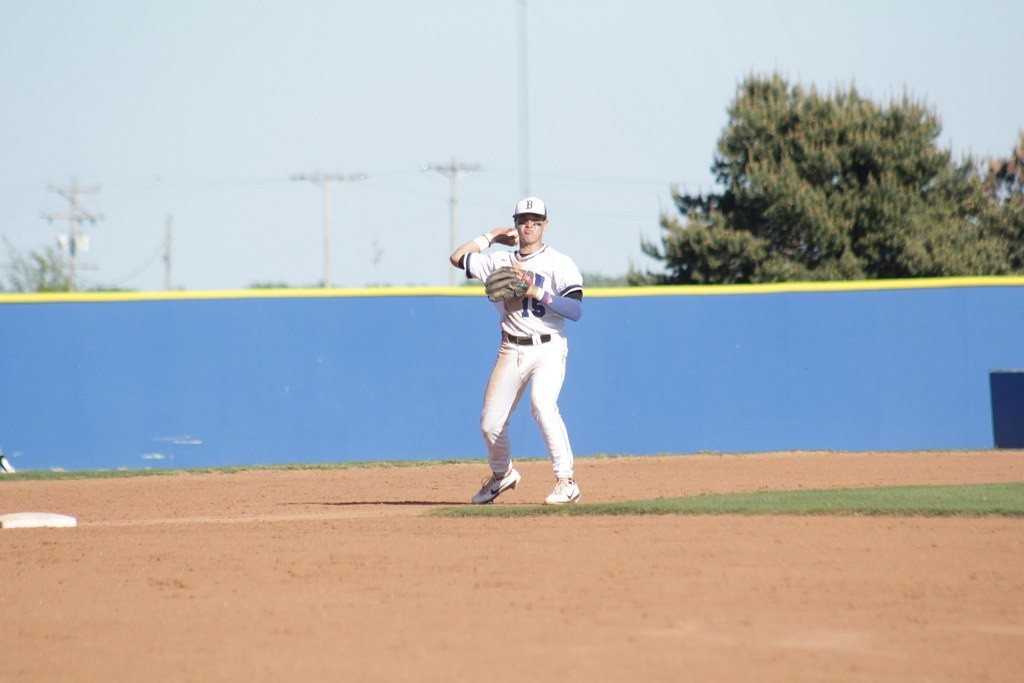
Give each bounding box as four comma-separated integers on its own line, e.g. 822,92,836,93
507,231,519,243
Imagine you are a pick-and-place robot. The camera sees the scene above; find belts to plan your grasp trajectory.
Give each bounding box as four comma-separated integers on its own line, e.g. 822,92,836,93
501,330,551,346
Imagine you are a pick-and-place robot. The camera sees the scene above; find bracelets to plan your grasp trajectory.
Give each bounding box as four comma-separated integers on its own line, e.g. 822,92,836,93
534,286,543,300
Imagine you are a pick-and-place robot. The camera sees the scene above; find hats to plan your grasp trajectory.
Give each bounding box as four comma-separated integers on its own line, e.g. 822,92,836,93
512,197,547,220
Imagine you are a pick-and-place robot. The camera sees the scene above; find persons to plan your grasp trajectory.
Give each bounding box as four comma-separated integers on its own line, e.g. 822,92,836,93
449,198,584,504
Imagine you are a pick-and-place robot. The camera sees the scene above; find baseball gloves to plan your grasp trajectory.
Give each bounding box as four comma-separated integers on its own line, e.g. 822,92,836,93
484,265,534,302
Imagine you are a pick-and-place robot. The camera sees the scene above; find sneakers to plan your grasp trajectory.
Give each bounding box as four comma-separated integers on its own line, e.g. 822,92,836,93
544,478,581,505
471,469,521,504
